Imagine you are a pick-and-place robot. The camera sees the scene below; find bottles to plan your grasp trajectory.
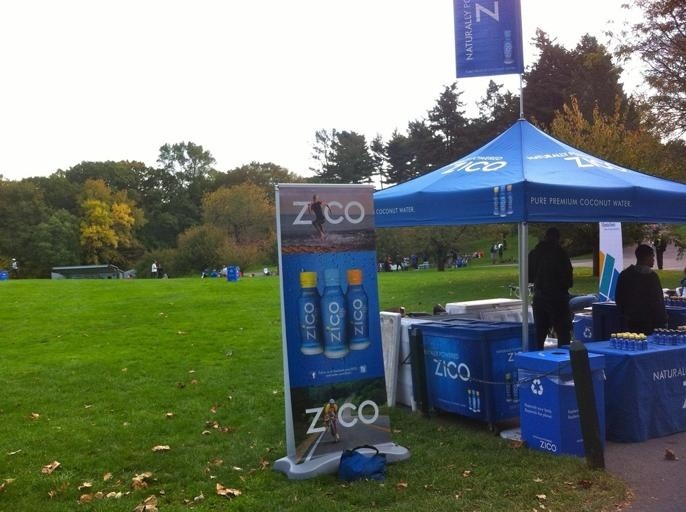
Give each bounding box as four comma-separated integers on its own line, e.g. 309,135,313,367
296,269,368,362
609,325,686,351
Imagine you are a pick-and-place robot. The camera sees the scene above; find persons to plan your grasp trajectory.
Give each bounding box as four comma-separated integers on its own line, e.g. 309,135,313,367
377,245,484,271
498,242,503,257
654,234,667,269
529,228,573,349
201,265,240,278
12,258,18,278
616,245,669,335
308,195,332,242
491,241,498,264
152,260,168,279
326,399,340,440
641,237,652,247
264,264,269,275
680,268,686,286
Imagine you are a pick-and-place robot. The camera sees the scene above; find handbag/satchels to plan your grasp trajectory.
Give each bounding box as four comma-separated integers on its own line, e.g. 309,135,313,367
337,444,386,481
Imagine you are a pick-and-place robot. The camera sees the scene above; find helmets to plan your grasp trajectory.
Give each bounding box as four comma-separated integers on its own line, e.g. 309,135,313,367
329,399,336,404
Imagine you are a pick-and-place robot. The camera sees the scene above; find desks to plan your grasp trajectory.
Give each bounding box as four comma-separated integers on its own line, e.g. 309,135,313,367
584,297,686,444
